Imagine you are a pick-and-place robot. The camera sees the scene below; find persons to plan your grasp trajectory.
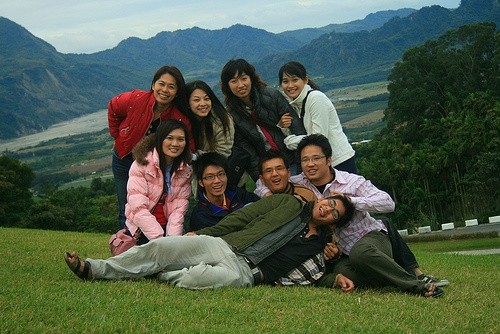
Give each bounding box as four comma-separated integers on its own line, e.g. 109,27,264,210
182,152,260,235
258,148,450,288
219,59,306,185
108,66,194,241
65,194,355,291
184,81,249,193
275,60,359,175
123,118,193,246
253,133,443,299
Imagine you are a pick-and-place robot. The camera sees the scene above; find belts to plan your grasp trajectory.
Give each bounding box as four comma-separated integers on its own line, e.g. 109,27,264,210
244,256,261,286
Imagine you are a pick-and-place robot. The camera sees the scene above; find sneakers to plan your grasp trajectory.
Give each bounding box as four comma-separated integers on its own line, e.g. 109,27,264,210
417,273,450,287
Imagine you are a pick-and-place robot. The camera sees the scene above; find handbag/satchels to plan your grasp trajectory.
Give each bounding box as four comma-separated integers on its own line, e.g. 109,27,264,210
109,228,137,257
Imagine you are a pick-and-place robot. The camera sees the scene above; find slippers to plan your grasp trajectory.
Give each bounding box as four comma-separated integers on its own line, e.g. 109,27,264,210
423,284,444,297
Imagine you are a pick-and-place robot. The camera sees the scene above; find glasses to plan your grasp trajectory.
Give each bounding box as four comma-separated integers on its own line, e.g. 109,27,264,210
302,155,326,163
202,171,226,181
328,198,339,221
262,166,287,175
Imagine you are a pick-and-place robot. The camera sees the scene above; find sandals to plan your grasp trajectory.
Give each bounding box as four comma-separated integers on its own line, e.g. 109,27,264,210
63,252,91,283
